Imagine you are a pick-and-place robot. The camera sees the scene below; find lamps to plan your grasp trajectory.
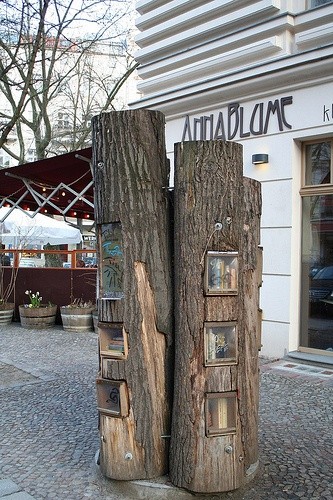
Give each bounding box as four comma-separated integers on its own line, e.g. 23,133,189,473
252,154,268,165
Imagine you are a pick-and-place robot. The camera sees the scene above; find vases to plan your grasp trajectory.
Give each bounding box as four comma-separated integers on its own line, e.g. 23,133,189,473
60,306,93,332
19,304,58,328
0,303,14,326
91,308,98,334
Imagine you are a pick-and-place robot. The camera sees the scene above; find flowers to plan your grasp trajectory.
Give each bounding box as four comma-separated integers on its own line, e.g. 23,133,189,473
0,299,7,304
62,299,93,309
24,290,54,309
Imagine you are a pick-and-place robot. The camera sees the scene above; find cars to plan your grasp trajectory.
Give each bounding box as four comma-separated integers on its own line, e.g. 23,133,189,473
308,264,333,318
83,257,97,268
